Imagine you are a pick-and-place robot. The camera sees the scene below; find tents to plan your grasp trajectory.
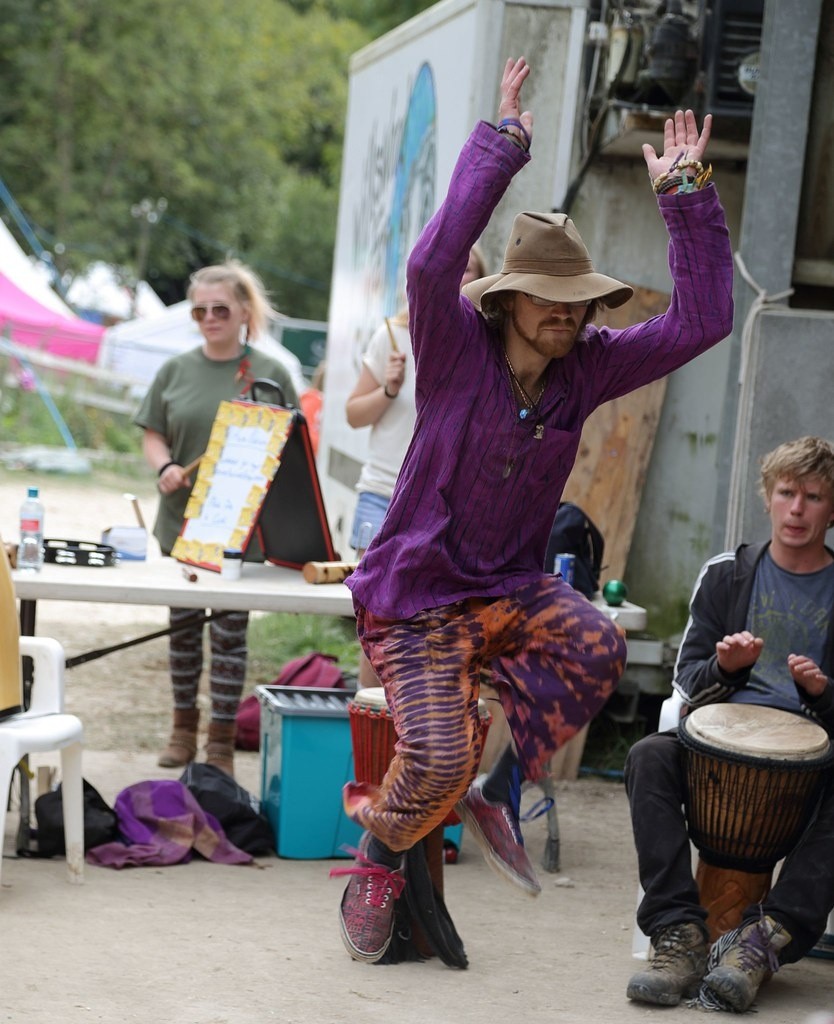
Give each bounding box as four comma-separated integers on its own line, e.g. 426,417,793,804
99,291,300,393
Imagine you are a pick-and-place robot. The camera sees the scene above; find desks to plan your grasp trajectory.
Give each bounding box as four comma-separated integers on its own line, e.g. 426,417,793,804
12,556,649,875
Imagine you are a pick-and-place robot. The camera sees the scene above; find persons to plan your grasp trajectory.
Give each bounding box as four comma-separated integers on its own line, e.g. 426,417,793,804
346,241,486,693
337,56,734,965
130,266,301,781
622,436,834,1015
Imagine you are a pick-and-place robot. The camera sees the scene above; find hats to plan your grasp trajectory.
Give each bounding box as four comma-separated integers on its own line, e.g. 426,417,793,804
461,212,634,313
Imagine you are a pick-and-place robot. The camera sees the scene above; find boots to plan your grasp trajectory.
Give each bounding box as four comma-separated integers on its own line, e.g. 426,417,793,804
158,708,199,767
204,721,236,779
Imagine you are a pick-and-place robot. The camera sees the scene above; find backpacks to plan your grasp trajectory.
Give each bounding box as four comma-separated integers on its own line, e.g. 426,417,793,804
234,653,345,747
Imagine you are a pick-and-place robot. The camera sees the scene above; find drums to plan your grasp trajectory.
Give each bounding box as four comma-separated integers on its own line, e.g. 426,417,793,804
346,685,493,958
675,701,831,952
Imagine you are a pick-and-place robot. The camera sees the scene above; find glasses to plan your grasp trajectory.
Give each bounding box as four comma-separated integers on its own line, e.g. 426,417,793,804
191,305,244,321
523,292,593,306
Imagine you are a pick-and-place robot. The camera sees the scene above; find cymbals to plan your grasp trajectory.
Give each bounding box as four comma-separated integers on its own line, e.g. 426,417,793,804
44,537,122,567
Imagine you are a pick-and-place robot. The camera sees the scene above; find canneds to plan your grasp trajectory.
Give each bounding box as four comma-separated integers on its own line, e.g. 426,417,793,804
554,553,575,587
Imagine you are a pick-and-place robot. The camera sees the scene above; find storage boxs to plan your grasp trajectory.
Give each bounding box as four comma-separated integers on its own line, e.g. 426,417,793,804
254,685,465,859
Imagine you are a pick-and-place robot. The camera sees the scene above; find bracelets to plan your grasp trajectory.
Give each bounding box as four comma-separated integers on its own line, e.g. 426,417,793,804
157,461,184,477
385,385,399,398
493,118,531,155
653,151,713,195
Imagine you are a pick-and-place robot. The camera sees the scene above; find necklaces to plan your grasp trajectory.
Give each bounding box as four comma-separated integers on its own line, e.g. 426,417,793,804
503,350,545,479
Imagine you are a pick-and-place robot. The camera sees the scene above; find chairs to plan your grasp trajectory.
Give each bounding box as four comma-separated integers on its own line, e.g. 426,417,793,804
0,636,85,886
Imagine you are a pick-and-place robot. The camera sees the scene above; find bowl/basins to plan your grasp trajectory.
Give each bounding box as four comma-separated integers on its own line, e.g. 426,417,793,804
103,525,149,560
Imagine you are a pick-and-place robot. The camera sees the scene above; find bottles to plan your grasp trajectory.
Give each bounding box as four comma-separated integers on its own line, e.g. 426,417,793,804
223,549,243,581
16,488,44,573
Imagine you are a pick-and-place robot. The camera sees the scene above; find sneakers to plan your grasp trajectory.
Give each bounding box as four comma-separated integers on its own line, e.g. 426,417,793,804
705,899,793,1011
329,831,406,964
627,924,708,1006
453,773,555,899
